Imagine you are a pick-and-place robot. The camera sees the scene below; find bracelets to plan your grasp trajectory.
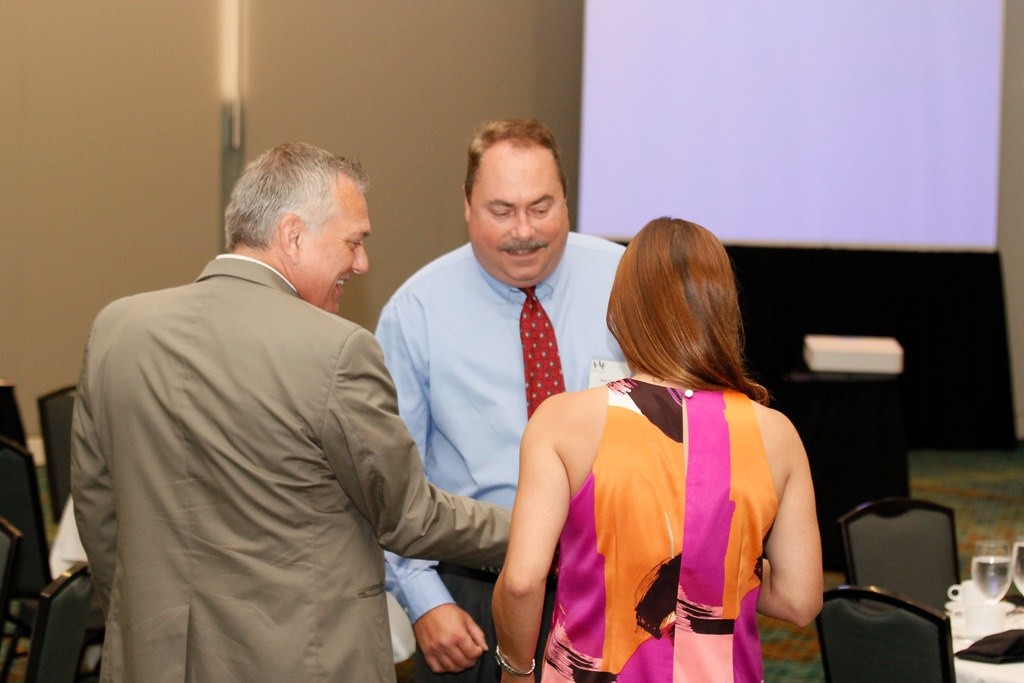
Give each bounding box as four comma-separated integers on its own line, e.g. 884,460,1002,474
495,645,535,677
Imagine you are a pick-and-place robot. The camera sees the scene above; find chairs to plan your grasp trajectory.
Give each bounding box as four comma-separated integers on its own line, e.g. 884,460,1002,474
838,497,962,613
0,379,27,451
816,584,956,683
0,435,51,683
0,517,22,593
24,565,93,683
37,385,76,520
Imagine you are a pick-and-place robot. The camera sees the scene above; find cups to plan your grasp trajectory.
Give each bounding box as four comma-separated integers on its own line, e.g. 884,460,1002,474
1012,535,1024,596
972,540,1013,606
948,580,984,608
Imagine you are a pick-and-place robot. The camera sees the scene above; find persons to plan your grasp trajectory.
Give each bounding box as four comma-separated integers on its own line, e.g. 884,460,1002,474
493,217,823,683
49,120,630,683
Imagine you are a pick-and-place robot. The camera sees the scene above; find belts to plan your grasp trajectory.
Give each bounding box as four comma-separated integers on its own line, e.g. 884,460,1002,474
439,562,499,585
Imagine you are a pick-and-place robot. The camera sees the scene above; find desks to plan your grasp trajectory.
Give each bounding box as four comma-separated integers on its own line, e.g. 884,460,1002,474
946,592,1024,683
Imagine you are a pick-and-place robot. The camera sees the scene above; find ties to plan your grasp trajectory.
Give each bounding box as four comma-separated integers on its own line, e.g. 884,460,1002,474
519,285,567,421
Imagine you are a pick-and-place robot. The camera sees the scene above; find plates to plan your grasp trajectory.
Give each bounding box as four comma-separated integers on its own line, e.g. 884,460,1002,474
945,600,1015,613
